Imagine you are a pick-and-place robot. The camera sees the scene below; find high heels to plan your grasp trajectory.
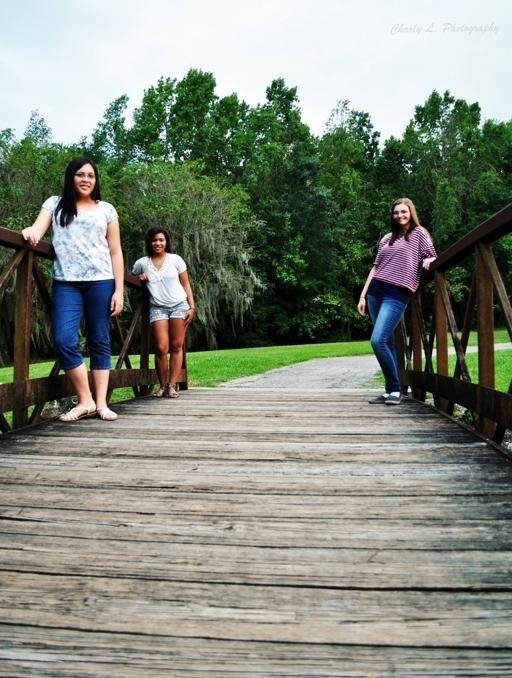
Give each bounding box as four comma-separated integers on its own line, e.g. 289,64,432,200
153,383,168,397
169,385,180,398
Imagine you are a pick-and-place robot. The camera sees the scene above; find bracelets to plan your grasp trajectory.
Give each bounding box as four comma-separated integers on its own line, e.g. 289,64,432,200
190,307,195,310
360,296,366,298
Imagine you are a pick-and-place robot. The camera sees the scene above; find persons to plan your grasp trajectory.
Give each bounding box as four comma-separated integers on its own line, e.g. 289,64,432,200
357,196,438,408
131,228,196,397
20,156,128,421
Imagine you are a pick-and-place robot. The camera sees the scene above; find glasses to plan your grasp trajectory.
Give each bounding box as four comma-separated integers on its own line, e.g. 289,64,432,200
72,173,95,180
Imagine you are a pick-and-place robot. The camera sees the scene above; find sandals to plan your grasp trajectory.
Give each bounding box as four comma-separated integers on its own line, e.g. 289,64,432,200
96,407,117,421
58,400,96,422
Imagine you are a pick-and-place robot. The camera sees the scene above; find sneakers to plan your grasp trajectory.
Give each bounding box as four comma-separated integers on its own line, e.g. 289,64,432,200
369,393,390,404
385,391,401,405
